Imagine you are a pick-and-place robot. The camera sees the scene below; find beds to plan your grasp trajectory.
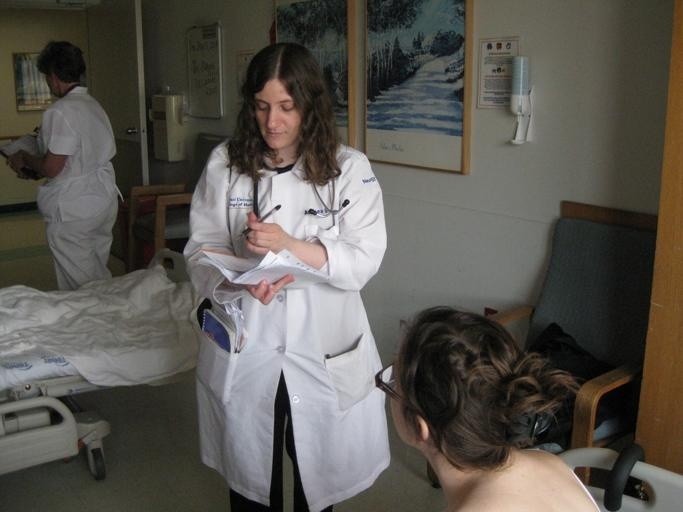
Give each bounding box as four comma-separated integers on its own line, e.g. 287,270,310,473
0,248,190,482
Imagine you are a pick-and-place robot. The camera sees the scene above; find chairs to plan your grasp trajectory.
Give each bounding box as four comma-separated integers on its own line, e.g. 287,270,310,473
426,202,658,490
126,133,234,271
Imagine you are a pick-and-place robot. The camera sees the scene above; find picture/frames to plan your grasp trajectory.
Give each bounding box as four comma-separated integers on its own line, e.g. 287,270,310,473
186,22,223,120
274,0,356,149
363,0,474,175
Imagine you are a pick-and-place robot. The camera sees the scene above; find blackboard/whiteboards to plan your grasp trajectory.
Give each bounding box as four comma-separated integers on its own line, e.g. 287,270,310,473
183,19,225,122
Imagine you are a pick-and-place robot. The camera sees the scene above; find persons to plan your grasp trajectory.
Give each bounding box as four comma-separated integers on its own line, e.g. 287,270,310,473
376,305,604,512
7,41,125,291
183,40,391,511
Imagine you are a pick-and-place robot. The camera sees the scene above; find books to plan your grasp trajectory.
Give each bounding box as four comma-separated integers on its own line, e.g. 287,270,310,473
195,309,236,401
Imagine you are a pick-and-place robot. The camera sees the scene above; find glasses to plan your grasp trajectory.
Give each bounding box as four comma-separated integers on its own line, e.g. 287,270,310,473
375,364,403,401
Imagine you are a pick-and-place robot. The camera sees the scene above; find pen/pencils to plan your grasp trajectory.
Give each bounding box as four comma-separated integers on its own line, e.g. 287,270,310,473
241,206,280,237
